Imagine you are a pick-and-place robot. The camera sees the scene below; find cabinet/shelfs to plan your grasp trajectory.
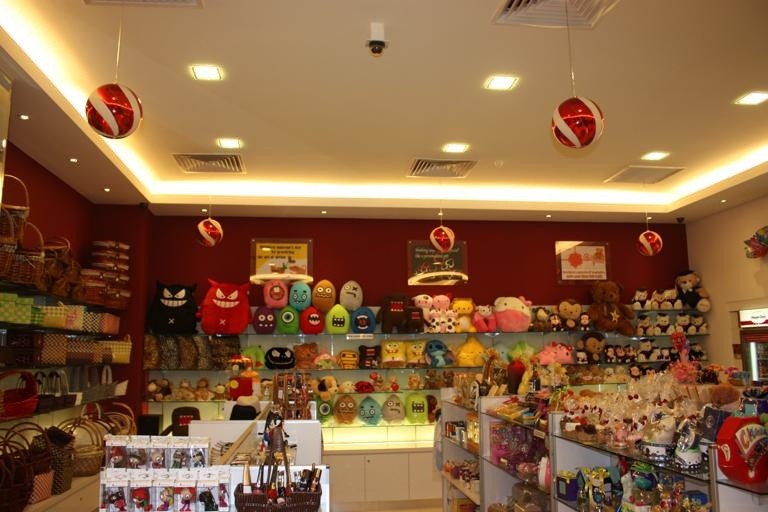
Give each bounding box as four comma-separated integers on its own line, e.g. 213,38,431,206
2,170,125,512
438,385,767,512
144,320,713,505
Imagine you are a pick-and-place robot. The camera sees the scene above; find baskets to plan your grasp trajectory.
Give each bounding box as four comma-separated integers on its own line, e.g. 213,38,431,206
234,483,321,512
0,366,128,420
0,403,137,512
0,174,88,300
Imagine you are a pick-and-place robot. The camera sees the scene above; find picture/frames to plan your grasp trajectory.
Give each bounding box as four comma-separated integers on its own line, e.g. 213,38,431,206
553,240,611,286
247,234,317,288
406,238,469,286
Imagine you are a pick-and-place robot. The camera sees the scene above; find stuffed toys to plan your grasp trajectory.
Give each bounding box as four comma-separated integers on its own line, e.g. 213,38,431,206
147,370,456,405
375,290,591,334
145,276,380,336
242,342,383,368
380,338,575,366
588,269,711,335
574,330,711,367
143,266,712,405
